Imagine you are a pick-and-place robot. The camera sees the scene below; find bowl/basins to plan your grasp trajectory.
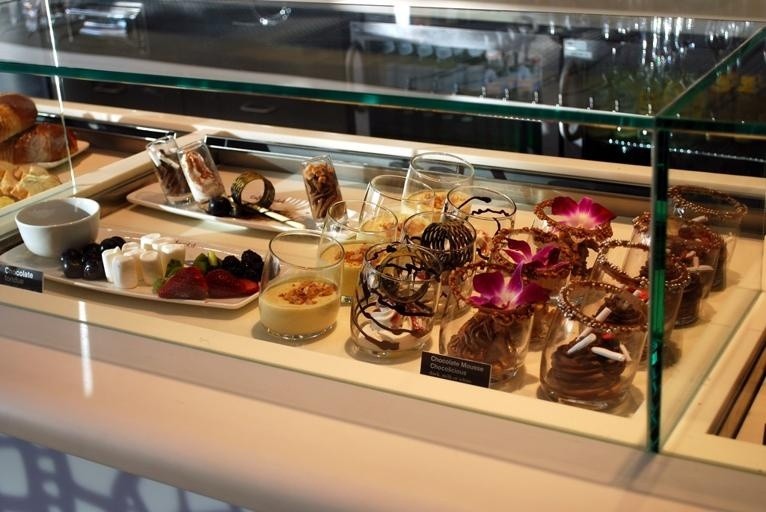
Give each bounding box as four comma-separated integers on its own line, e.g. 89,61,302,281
11,197,101,262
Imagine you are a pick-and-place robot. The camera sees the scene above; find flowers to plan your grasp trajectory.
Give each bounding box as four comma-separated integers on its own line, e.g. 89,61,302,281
499,233,574,277
466,260,556,313
551,194,619,230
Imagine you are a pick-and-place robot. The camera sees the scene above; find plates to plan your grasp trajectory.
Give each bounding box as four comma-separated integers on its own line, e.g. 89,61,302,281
124,172,373,244
1,227,276,313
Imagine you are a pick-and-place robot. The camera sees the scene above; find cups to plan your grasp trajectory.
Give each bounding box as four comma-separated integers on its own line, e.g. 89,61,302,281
438,185,519,274
358,175,438,244
402,154,476,229
662,182,744,287
259,230,340,343
319,198,396,305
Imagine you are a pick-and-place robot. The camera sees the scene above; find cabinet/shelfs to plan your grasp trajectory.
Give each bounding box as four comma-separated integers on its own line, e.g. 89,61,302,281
1,0,766,512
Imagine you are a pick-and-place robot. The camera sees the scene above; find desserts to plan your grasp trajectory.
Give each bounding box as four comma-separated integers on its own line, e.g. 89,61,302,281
437,185,517,264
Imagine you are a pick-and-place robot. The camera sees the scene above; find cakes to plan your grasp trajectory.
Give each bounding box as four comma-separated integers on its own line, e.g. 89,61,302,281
153,147,224,204
302,164,346,222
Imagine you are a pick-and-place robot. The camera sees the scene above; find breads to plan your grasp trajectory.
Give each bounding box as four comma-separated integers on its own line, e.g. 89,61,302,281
0,94,77,208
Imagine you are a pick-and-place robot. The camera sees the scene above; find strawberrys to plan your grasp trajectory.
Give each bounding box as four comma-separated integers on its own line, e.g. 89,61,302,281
157,267,208,298
206,269,260,298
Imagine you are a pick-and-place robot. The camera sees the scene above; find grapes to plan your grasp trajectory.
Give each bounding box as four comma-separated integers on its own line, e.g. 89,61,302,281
222,250,264,282
209,197,233,216
61,237,126,280
